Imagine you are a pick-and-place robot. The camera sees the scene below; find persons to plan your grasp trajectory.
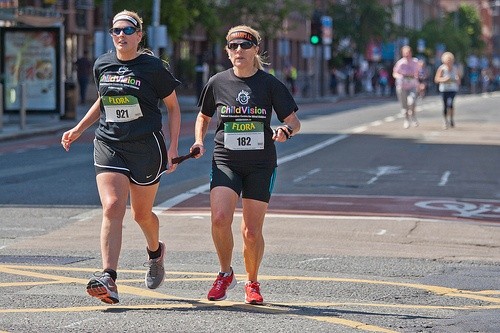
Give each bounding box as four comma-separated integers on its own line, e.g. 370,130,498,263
434,52,459,128
287,62,297,97
393,46,422,129
193,55,208,102
377,68,389,97
77,51,90,104
416,60,427,99
189,24,300,303
62,11,181,304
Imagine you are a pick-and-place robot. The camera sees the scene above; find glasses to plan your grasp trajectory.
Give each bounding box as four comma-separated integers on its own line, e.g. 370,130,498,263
228,42,257,50
109,27,138,37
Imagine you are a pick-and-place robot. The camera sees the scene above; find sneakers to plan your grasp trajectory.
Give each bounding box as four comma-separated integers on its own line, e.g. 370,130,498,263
244,281,264,305
87,272,120,303
208,268,237,301
143,241,166,289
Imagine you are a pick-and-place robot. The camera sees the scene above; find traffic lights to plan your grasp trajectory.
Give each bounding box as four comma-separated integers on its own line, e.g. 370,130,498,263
310,14,322,47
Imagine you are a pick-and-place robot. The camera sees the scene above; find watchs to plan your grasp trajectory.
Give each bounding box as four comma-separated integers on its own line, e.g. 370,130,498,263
283,125,293,135
